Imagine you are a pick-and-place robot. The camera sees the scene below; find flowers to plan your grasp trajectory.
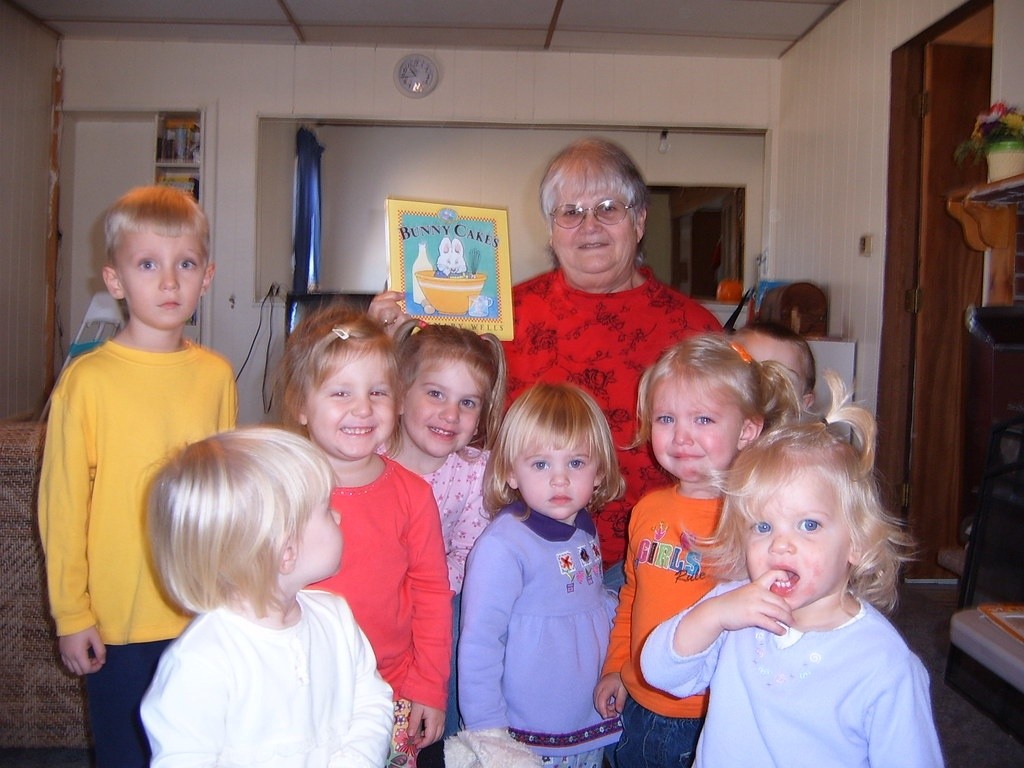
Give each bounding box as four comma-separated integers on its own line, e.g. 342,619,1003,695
950,101,1024,171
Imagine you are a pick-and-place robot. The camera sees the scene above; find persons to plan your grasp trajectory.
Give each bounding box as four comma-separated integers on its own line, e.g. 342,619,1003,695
367,138,724,571
134,427,394,768
734,321,817,409
640,368,944,768
272,304,452,768
374,318,506,600
592,331,801,768
39,185,238,768
458,380,622,768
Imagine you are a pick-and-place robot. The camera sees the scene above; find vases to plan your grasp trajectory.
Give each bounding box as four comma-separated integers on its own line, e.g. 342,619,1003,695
983,140,1024,184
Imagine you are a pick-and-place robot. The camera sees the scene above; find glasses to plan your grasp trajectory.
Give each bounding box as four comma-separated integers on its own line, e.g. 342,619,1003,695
547,198,642,229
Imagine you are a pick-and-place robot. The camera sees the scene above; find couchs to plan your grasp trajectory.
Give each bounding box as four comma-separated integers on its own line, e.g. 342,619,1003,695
0,410,88,750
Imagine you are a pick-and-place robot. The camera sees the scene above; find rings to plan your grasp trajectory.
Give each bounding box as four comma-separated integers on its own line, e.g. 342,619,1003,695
384,319,387,327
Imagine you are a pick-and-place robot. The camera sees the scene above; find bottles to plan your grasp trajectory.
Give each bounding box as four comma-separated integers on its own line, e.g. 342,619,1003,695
984,138,1024,183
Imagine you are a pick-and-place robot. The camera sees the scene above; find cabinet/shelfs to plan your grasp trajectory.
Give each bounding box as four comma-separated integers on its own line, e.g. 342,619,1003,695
154,111,202,346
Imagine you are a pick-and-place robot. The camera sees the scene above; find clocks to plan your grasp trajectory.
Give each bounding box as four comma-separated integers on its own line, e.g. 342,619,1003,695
393,55,438,98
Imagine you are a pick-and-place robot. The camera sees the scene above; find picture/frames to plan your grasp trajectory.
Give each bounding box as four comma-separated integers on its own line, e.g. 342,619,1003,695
385,197,515,341
